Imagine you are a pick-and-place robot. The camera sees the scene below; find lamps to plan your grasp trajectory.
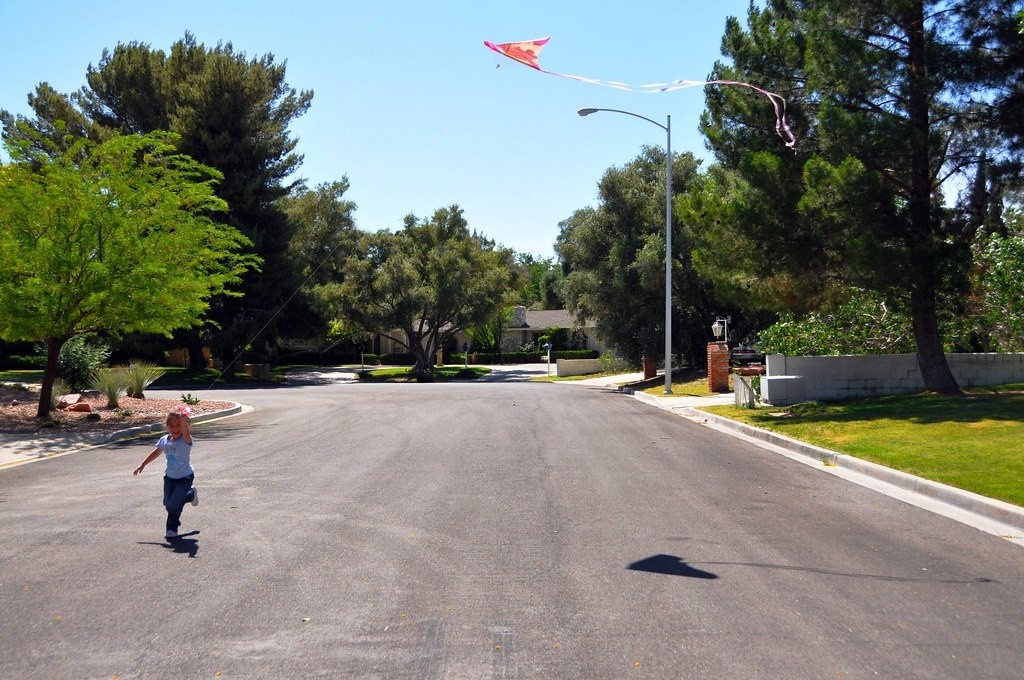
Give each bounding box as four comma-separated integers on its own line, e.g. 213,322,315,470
711,321,724,339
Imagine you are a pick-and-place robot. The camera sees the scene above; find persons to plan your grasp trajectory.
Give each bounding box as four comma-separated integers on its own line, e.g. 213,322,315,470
134,405,199,537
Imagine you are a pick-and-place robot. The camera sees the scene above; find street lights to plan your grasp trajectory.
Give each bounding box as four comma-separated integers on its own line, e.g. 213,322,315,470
578,108,672,394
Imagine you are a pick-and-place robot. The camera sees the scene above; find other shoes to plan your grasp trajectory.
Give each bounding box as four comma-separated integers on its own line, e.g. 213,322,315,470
191,486,198,506
165,529,178,537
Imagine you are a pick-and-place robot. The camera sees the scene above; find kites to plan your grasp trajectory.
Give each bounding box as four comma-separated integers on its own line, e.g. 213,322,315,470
484,36,798,155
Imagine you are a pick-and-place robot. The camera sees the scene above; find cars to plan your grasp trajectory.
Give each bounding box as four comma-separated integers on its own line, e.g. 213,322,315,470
733,340,767,366
